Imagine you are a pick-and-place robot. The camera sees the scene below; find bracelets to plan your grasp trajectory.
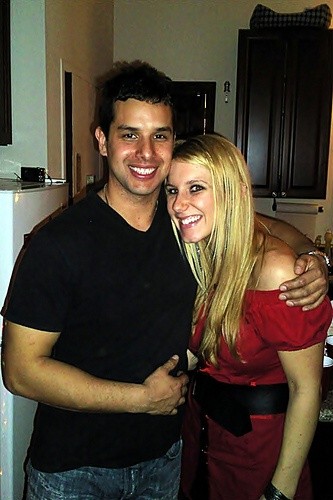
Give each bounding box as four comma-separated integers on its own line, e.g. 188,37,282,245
263,481,291,500
308,250,331,274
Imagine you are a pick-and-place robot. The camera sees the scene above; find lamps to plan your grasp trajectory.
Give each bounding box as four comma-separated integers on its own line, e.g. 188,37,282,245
223,80,231,104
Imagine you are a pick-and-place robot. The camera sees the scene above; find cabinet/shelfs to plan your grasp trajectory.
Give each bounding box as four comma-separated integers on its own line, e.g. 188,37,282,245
234,28,332,199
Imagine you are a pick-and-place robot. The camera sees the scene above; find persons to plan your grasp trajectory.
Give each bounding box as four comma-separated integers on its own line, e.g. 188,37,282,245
163,132,333,500
0,61,332,500
